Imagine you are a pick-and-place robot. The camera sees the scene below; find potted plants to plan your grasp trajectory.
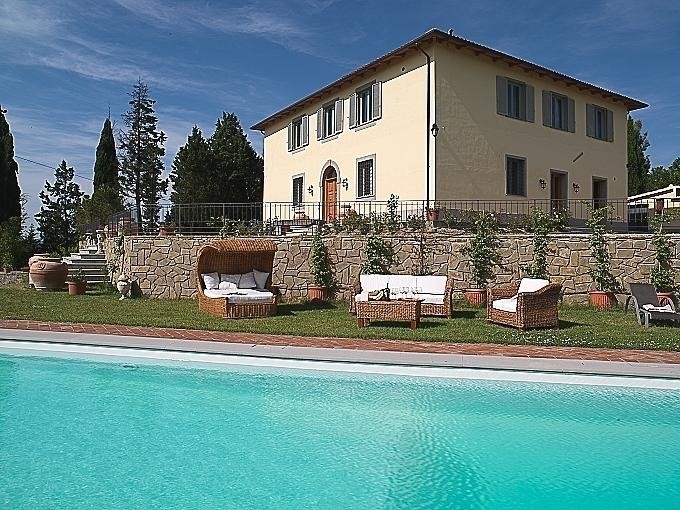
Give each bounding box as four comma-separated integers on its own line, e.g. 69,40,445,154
308,231,330,302
65,267,87,296
647,209,680,307
460,212,503,307
584,208,625,310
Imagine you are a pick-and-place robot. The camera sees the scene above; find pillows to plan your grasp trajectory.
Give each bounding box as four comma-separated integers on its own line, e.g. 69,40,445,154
201,269,270,290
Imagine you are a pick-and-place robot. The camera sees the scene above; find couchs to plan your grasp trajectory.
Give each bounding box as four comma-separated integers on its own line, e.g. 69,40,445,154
349,275,454,319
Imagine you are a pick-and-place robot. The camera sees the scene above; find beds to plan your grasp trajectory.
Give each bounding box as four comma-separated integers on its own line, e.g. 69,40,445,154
195,240,279,319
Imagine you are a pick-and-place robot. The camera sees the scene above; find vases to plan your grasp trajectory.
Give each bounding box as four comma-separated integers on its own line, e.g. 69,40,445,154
427,210,439,221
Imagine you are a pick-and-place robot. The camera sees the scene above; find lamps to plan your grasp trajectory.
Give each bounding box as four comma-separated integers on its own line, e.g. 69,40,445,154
431,123,439,137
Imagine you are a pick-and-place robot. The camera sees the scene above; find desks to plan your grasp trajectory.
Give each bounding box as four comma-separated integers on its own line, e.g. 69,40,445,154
356,298,425,328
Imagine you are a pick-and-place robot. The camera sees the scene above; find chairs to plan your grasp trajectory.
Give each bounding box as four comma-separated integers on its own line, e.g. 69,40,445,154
625,283,680,328
486,280,563,331
